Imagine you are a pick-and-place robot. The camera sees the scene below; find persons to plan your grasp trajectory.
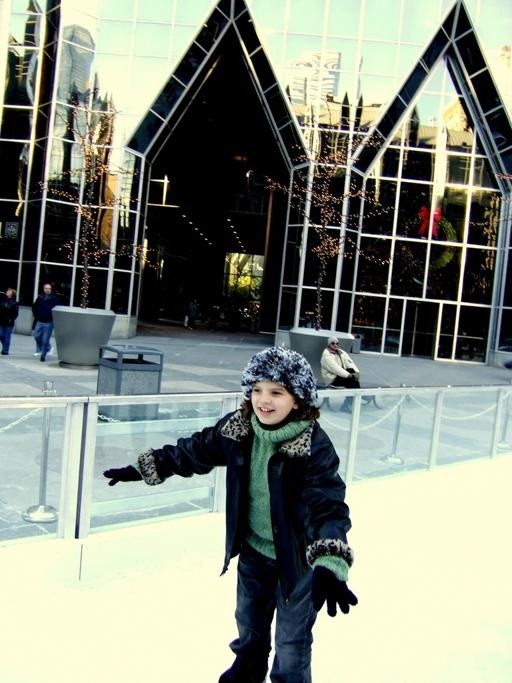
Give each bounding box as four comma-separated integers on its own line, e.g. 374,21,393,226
0,288,19,355
32,283,60,361
208,305,220,334
187,299,199,330
103,346,357,683
320,336,360,388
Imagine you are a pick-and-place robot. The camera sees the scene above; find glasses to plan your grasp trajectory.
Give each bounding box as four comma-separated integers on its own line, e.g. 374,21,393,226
331,343,338,345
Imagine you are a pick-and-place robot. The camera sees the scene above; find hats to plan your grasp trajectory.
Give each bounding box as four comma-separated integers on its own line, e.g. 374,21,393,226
240,345,319,407
328,335,339,345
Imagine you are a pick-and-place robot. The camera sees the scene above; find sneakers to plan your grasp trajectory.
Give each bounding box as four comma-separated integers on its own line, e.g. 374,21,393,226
33,352,45,361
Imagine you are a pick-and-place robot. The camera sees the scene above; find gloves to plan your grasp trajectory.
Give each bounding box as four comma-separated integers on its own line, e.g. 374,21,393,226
310,555,358,617
103,462,145,486
349,372,360,381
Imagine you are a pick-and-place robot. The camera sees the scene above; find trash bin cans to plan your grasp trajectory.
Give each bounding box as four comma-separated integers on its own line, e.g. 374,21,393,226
96,344,163,422
